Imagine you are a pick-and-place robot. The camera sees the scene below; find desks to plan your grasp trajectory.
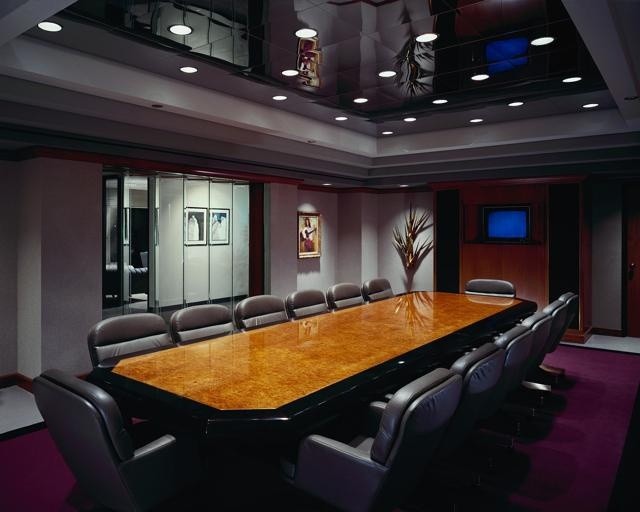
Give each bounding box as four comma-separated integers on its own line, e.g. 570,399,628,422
88,290,537,442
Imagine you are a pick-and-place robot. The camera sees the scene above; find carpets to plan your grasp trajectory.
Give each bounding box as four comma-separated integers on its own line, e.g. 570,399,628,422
1,342,638,510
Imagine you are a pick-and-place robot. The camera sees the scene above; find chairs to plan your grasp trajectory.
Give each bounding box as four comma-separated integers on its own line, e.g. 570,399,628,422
170,304,233,344
31,369,176,511
520,300,568,325
384,343,505,419
234,296,288,329
363,278,393,301
558,291,579,328
88,313,172,365
500,311,568,390
464,279,515,297
294,368,463,511
460,325,553,417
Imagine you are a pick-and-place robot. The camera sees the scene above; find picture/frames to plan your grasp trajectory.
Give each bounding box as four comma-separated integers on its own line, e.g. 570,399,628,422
184,207,207,245
327,284,363,309
285,290,327,317
297,212,321,258
210,209,229,244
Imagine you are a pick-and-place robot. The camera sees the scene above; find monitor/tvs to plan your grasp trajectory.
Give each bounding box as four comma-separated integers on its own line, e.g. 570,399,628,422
479,204,533,244
487,38,530,77
